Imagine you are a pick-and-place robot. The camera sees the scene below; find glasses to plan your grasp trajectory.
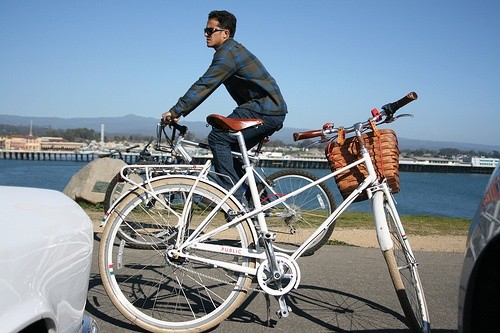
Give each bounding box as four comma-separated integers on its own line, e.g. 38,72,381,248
204,27,227,35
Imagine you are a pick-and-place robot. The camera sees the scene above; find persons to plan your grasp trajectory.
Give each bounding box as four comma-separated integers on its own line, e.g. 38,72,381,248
161,10,288,225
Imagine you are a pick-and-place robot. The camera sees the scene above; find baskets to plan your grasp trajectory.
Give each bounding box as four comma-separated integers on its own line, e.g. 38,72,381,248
325,129,401,203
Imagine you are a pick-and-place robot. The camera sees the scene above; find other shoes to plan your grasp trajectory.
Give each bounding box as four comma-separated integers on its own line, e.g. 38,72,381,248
224,202,274,223
200,195,242,208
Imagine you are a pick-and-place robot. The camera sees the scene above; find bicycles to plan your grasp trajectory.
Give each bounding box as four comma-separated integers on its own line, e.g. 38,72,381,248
103,115,338,258
97,87,430,333
